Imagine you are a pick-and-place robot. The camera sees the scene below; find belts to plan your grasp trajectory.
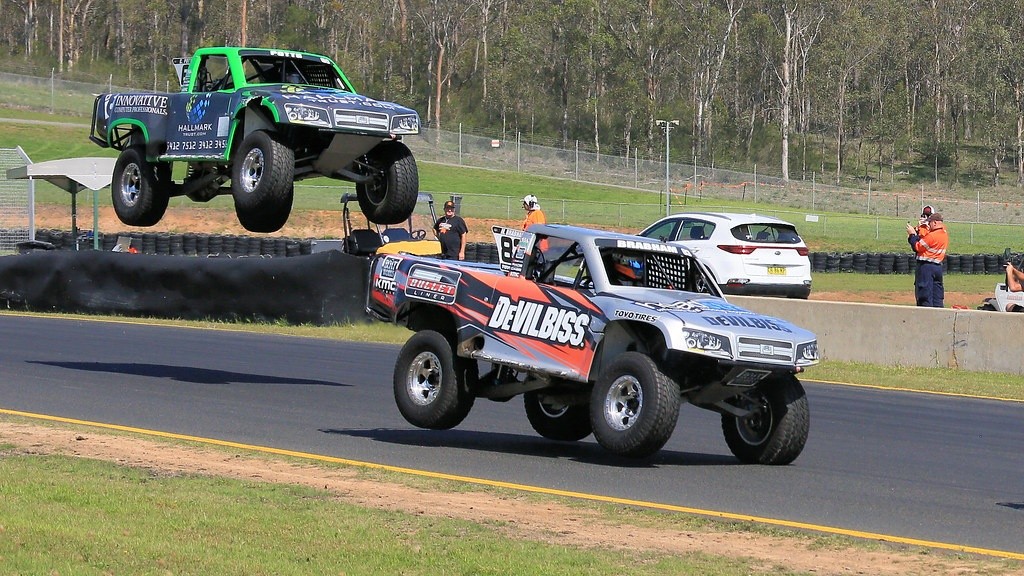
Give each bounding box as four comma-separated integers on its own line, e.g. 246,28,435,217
917,260,932,264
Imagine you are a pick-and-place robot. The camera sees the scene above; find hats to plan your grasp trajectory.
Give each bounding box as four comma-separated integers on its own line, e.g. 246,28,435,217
923,206,932,213
925,213,943,221
443,201,456,209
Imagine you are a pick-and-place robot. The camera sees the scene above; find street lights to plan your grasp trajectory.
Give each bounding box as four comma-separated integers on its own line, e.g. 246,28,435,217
656,119,680,217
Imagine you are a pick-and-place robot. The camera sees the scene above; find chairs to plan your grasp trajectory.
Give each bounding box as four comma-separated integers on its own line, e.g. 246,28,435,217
349,229,382,255
383,228,413,244
689,226,706,240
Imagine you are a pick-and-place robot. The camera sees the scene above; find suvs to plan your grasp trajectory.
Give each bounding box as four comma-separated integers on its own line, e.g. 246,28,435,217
634,212,812,300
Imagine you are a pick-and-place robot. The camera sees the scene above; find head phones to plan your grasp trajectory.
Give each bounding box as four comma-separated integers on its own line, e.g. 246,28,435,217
529,195,534,208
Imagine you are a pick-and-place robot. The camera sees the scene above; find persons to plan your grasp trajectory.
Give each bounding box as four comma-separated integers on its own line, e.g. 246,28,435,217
1006,261,1024,312
432,201,469,261
906,205,948,308
519,195,549,252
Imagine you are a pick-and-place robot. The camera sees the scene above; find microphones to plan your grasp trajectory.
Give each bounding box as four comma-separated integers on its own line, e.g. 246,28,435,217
522,207,524,208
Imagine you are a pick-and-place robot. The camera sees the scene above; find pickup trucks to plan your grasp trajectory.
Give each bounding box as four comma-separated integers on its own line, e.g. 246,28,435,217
366,222,824,466
89,47,422,234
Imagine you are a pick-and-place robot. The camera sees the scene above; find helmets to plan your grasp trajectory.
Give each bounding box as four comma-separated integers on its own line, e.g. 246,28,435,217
520,194,537,208
611,248,644,281
